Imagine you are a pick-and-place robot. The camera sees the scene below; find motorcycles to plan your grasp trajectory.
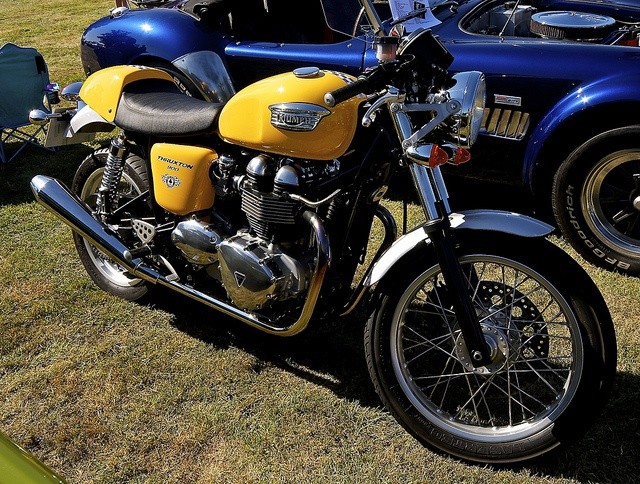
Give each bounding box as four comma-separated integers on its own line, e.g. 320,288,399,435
29,28,617,469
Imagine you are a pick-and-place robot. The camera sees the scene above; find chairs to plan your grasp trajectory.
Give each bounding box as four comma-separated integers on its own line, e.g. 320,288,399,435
0,44,52,163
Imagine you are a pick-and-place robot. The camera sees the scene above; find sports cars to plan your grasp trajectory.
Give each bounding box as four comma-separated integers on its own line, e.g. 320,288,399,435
81,1,640,276
